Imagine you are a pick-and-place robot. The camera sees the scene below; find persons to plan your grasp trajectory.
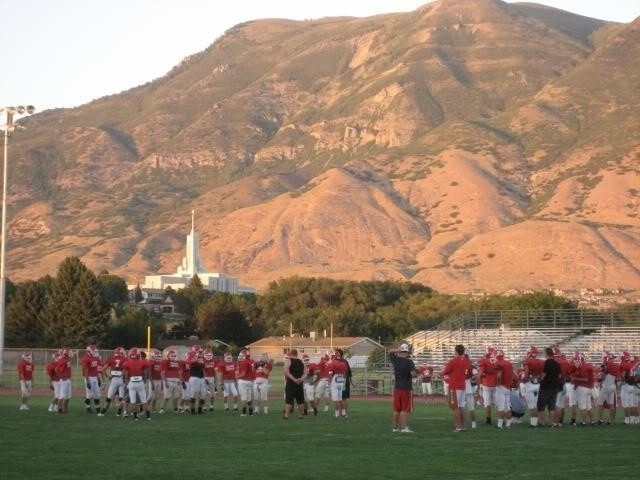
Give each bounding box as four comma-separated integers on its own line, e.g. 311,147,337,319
418,361,434,404
389,342,418,435
80,345,274,423
284,347,352,418
18,350,35,412
442,343,640,430
47,347,73,412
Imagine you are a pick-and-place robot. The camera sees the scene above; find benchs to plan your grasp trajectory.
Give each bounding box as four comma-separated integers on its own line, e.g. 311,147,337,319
373,326,640,385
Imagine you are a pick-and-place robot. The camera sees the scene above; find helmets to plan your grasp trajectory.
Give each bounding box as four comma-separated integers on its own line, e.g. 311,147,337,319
486,346,630,367
285,351,341,365
22,345,268,363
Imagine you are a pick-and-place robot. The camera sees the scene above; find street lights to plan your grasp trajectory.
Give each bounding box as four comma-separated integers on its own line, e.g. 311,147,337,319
0,103,35,365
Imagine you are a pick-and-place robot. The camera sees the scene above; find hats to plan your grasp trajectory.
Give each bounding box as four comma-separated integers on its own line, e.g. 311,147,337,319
400,344,409,352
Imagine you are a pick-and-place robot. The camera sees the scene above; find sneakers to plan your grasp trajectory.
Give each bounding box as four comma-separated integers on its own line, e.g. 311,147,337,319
454,421,639,433
20,404,268,422
393,428,414,434
283,408,349,420
